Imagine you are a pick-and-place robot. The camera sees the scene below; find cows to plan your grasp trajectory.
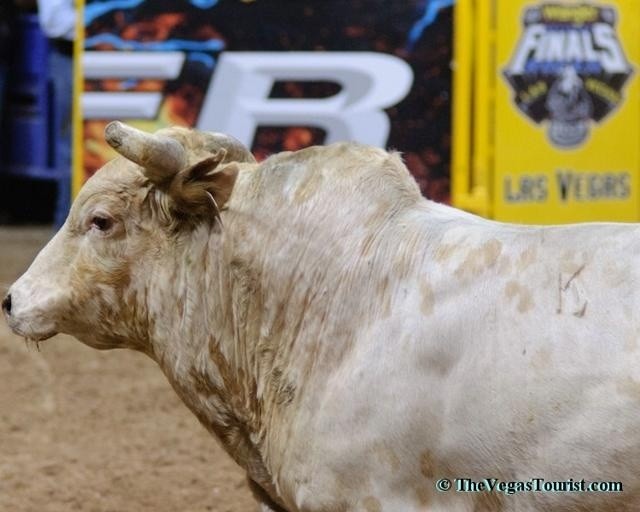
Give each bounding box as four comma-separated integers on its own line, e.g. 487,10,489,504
0,119,639,512
